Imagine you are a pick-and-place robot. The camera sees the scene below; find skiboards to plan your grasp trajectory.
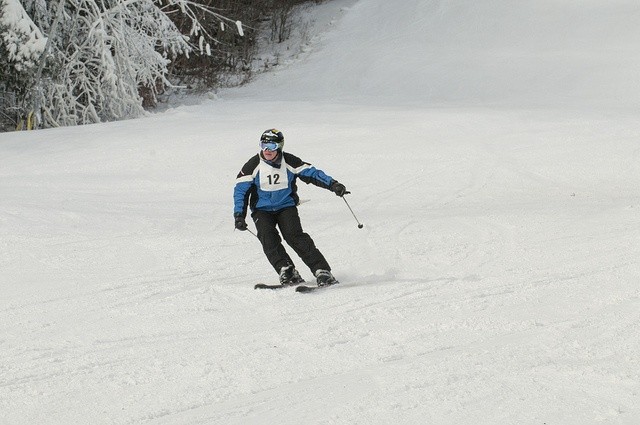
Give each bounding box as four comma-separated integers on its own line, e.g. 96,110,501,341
255,280,341,294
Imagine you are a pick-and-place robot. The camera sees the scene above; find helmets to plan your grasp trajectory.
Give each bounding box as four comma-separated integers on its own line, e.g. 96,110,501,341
260,129,284,152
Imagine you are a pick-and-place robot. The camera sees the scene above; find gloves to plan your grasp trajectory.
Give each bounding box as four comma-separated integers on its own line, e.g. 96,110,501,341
330,181,346,197
234,217,248,231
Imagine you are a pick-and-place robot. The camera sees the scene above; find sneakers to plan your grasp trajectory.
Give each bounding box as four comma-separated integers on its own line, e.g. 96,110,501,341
278,265,303,286
315,269,336,286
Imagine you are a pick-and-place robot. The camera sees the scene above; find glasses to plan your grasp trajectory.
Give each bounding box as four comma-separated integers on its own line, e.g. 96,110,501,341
259,142,280,151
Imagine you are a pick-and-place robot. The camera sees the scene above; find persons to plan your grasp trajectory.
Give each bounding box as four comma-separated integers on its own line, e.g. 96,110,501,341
231,127,351,287
